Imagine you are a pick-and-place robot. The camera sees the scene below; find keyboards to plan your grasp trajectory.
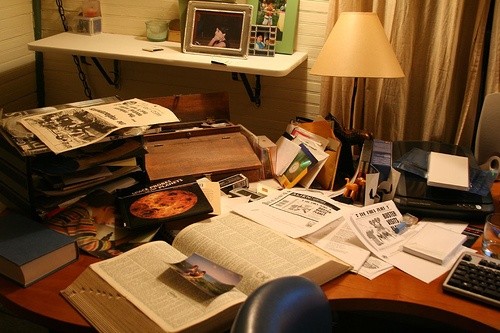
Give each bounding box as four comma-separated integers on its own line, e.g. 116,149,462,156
442,250,500,308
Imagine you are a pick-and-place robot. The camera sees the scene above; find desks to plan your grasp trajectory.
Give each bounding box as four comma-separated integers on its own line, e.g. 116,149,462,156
0,178,500,333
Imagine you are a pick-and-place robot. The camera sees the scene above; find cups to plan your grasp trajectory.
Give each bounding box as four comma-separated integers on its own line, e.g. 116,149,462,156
481,213,500,259
145,20,168,42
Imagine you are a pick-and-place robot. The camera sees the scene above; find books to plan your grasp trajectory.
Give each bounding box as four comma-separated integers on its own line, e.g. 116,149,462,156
0,95,471,333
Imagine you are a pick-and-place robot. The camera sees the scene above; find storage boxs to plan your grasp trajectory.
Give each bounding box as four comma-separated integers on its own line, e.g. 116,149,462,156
139,90,262,183
73,16,102,35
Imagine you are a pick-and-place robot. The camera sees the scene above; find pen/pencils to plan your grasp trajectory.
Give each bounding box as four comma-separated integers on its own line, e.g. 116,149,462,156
211,61,226,65
142,49,164,52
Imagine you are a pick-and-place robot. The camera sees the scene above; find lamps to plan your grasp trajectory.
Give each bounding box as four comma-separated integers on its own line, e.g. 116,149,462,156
309,11,405,131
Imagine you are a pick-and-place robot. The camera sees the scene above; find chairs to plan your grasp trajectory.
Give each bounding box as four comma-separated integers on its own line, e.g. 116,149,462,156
230,276,332,333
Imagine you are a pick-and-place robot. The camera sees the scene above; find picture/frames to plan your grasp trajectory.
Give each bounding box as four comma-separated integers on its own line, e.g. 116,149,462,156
245,0,299,55
183,1,253,57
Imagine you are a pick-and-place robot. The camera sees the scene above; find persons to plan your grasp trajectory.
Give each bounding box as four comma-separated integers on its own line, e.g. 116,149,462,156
45,189,134,253
208,24,227,48
256,32,266,49
259,0,285,24
186,264,206,280
